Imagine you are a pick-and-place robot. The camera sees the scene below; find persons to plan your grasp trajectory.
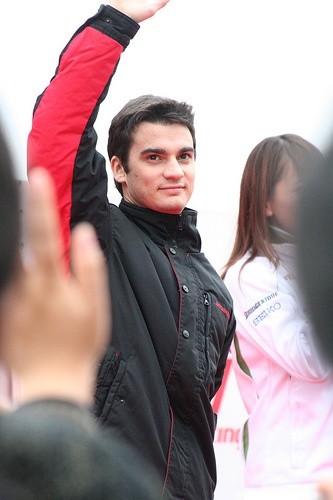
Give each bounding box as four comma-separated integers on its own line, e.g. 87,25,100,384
0,128,170,500
28,0,236,500
209,132,333,500
